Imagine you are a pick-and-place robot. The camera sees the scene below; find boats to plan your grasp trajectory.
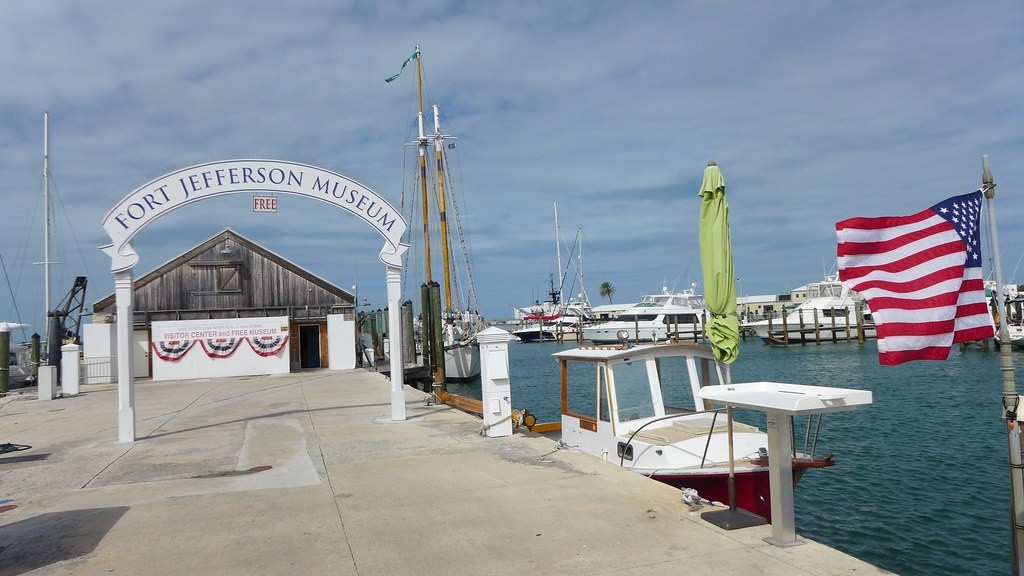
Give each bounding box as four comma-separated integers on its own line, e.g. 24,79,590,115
795,261,855,303
583,304,710,343
546,338,831,525
643,277,708,307
740,296,872,342
994,296,1024,350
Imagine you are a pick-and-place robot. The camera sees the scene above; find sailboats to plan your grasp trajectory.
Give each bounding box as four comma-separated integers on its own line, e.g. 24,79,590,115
509,203,587,339
385,74,486,379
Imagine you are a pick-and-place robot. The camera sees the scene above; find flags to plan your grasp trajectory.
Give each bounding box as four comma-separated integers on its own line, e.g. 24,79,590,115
835,190,997,366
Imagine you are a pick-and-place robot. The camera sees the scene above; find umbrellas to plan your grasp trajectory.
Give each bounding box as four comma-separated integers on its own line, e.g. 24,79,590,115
698,160,740,514
